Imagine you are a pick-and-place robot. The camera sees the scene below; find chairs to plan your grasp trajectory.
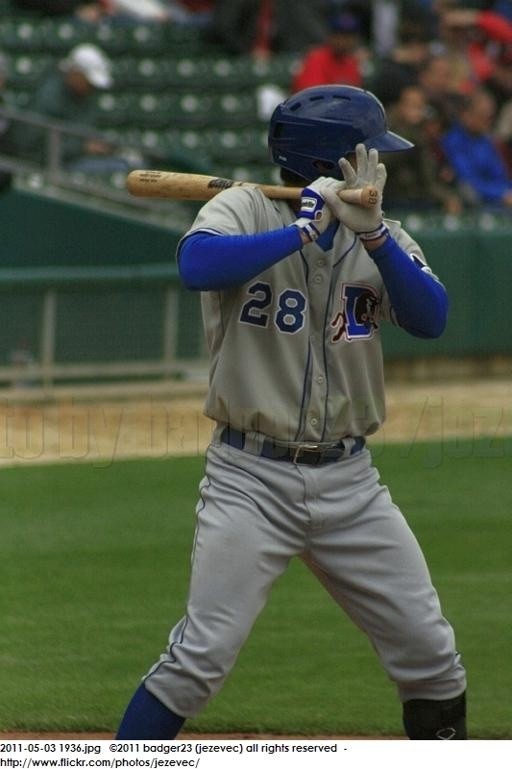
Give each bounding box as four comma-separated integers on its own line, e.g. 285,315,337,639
1,13,512,205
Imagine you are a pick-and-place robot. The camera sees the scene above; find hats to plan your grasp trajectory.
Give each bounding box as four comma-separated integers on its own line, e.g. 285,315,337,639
70,43,114,90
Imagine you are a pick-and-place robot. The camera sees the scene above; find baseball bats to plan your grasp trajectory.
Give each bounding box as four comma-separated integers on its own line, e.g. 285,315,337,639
126,169,378,208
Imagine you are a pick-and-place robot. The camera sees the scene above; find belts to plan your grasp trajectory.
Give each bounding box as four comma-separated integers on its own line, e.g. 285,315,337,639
221,427,366,466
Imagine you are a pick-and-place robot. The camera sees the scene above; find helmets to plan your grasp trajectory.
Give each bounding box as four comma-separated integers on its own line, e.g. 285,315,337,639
269,84,415,182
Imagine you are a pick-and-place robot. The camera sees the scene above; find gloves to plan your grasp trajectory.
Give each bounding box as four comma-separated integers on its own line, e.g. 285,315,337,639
289,144,388,242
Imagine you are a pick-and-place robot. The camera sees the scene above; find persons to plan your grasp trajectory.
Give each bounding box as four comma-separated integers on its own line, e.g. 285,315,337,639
116,84,468,740
203,0,512,211
2,43,146,178
